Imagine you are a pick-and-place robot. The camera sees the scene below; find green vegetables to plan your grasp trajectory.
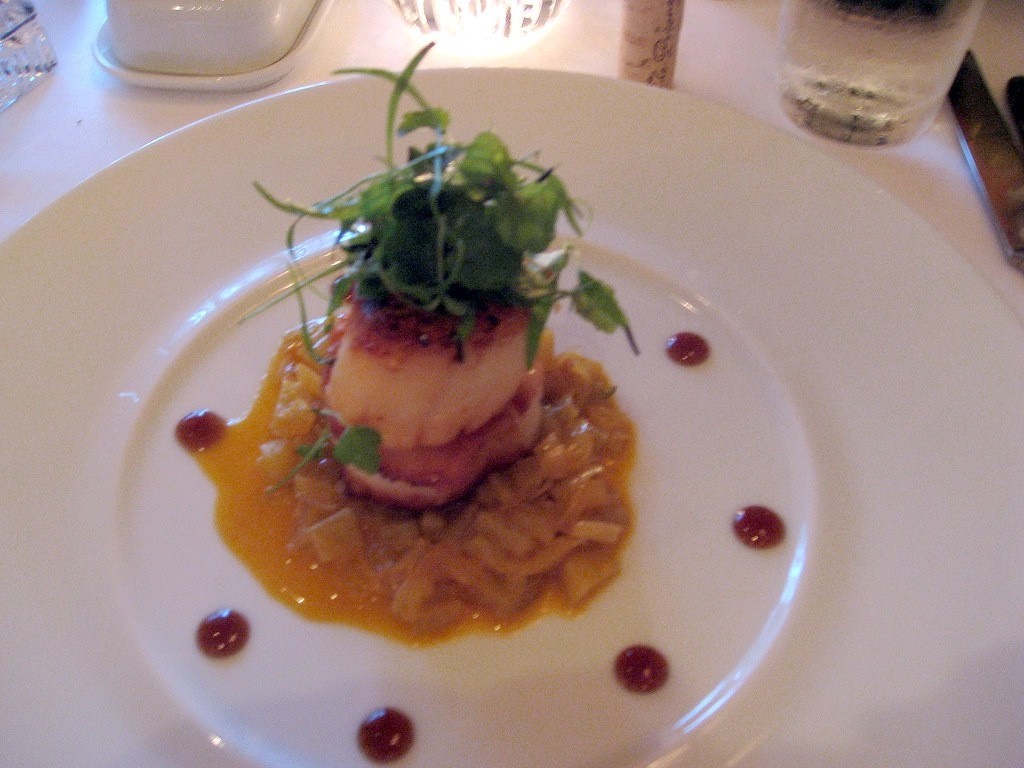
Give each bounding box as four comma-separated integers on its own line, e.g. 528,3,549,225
265,406,381,491
250,41,642,367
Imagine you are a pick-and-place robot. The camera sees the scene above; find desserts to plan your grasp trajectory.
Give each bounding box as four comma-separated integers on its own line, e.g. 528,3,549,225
326,299,544,507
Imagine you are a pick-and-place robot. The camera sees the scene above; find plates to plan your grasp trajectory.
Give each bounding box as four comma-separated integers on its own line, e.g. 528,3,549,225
0,62,1024,768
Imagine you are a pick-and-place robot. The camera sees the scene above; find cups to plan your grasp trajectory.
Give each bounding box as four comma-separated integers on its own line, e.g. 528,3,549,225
778,0,992,146
